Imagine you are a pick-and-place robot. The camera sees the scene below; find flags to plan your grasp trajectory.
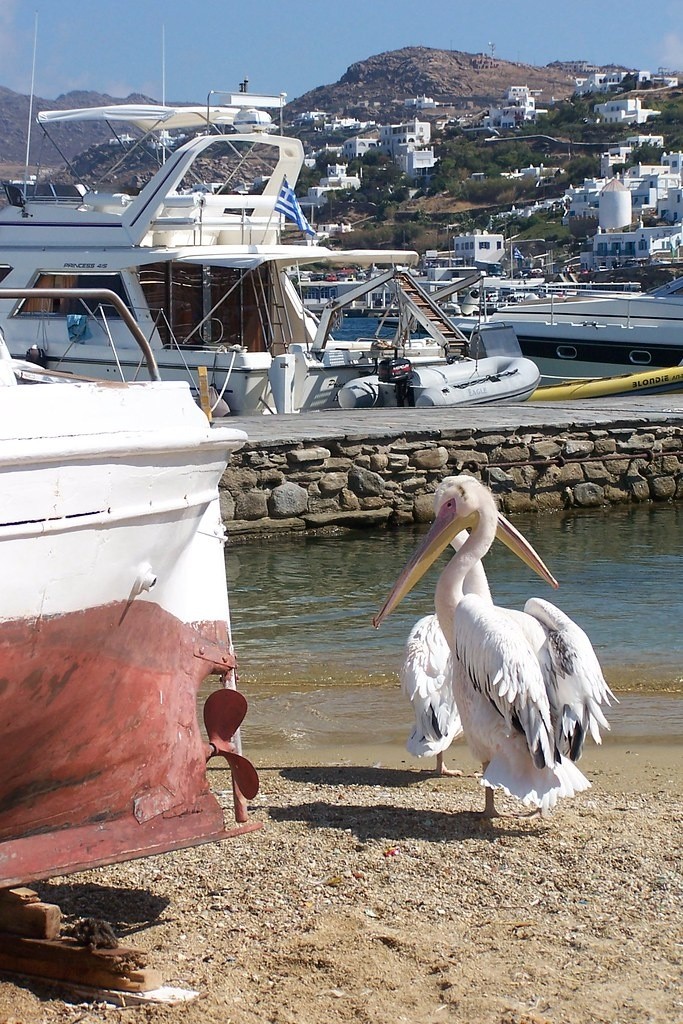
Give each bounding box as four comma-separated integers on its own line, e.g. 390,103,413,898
275,176,317,236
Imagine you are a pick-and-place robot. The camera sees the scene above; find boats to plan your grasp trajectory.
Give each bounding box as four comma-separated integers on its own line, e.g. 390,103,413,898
337,345,541,410
525,359,683,401
2,9,523,421
0,285,263,892
292,228,682,379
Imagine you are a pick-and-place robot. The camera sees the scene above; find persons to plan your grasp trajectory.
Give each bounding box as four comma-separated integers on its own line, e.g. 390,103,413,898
477,292,526,312
513,259,647,277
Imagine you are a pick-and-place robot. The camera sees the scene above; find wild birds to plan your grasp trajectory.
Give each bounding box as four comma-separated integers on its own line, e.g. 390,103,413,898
371,475,620,820
